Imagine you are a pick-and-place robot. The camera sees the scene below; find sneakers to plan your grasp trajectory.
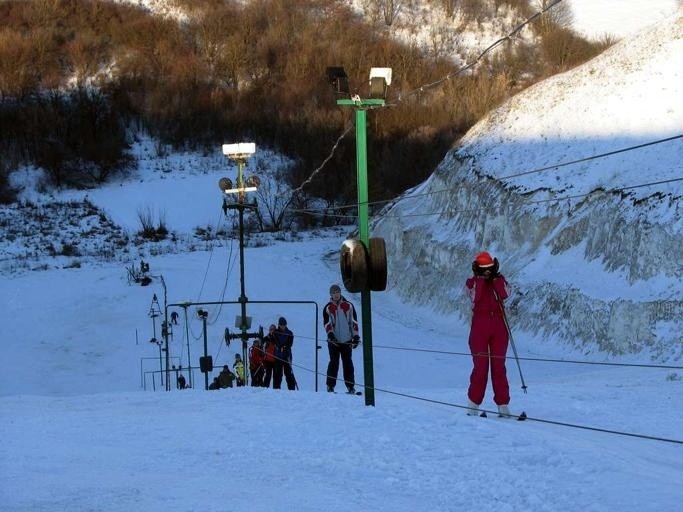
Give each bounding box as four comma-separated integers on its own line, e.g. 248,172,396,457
498,405,510,416
347,387,355,393
327,386,334,392
467,400,478,415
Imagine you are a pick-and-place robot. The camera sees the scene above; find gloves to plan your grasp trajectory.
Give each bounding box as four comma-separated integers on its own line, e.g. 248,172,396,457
328,332,337,343
472,261,479,275
352,335,359,348
492,257,499,274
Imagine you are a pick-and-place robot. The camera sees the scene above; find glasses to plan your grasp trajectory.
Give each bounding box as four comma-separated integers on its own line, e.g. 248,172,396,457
479,268,493,272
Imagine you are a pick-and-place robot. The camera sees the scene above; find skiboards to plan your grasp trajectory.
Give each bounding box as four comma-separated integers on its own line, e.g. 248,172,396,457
467,411,527,421
328,392,362,395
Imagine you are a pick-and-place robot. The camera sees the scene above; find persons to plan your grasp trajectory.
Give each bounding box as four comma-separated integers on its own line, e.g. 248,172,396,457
321,284,362,395
217,364,235,388
207,376,220,390
231,352,249,387
248,339,265,386
464,251,511,418
177,373,185,390
270,316,294,390
160,321,166,339
169,310,178,325
260,323,280,388
139,260,144,270
144,262,149,273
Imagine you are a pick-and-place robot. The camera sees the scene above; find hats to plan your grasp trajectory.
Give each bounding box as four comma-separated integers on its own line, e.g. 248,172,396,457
279,317,286,324
235,354,240,358
476,252,494,267
330,285,340,294
